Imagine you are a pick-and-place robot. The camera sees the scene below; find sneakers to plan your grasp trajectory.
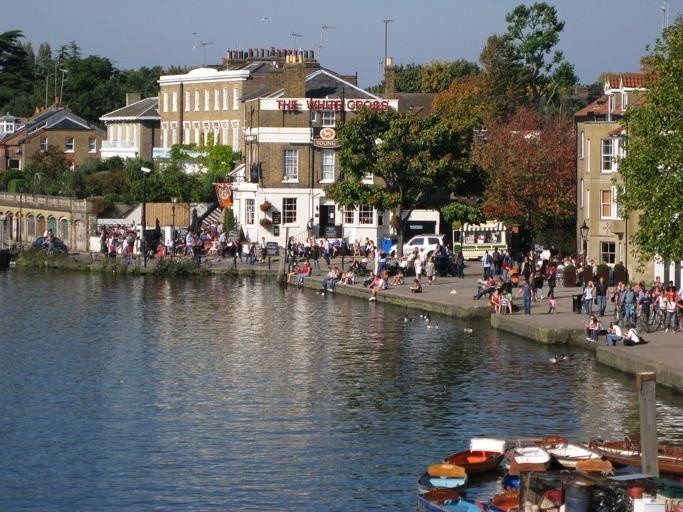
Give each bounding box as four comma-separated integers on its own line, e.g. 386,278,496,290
591,339,596,343
586,337,592,342
647,321,683,334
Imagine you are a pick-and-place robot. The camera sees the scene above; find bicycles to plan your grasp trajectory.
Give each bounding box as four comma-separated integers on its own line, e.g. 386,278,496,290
613,303,669,333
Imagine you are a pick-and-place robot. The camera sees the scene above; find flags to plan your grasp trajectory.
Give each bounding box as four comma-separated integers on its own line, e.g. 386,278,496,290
212,182,234,208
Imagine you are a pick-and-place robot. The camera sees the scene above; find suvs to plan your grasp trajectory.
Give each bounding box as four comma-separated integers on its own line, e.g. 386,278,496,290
388,234,450,258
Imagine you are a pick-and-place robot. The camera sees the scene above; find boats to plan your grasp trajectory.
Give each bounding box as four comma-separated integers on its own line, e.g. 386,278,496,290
415,432,682,511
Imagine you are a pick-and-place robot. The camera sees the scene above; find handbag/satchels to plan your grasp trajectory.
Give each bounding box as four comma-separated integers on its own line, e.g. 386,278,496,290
638,337,647,344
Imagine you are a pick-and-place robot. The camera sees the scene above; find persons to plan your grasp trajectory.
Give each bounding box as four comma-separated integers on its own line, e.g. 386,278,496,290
99,224,137,258
145,221,267,264
287,237,465,301
305,218,314,243
42,228,54,255
469,244,683,348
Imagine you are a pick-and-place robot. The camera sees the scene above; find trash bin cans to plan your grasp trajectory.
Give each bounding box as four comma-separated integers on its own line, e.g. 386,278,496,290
573,294,583,314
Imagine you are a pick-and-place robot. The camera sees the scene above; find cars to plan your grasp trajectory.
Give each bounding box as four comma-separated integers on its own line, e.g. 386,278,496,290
240,242,280,255
31,236,69,253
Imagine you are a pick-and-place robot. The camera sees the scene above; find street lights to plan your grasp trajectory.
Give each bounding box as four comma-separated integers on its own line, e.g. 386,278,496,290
579,220,590,267
620,112,628,284
168,192,178,257
